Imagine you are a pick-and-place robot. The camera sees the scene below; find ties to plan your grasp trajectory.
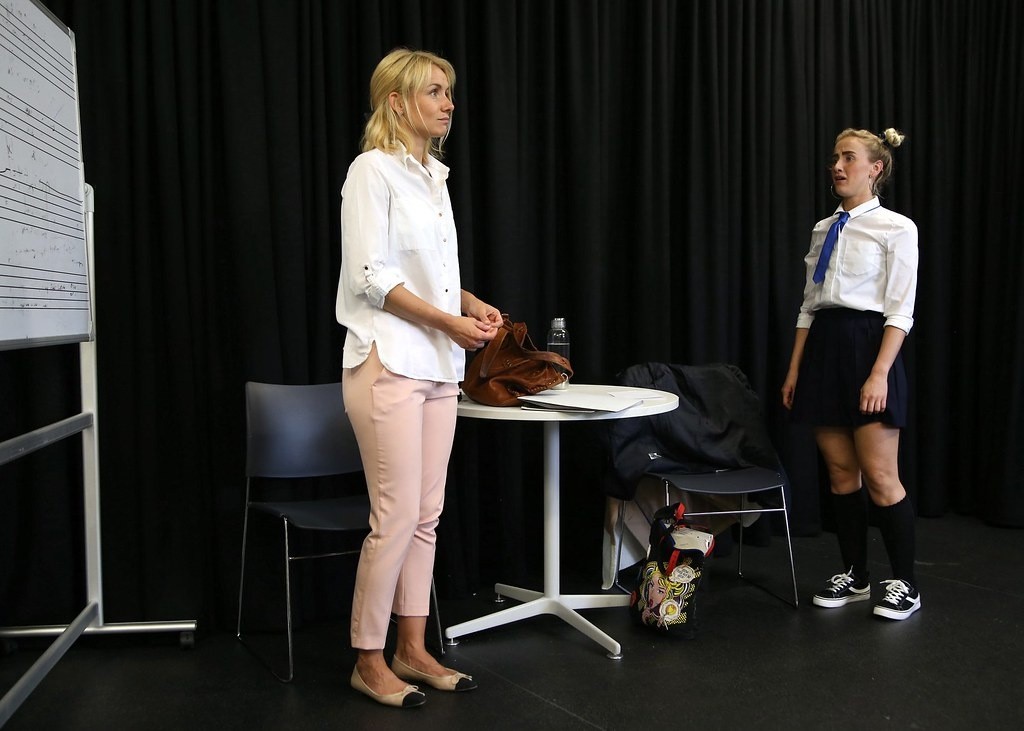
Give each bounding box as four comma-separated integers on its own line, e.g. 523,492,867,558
811,211,850,285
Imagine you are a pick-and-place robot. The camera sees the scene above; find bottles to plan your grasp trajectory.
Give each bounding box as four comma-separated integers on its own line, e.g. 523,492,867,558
547,318,569,390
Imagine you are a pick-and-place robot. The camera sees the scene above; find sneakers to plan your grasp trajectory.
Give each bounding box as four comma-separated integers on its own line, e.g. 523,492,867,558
811,564,871,609
872,576,922,621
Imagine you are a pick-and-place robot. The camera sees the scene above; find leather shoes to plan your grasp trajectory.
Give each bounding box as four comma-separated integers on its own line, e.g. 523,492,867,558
390,652,478,692
349,663,428,710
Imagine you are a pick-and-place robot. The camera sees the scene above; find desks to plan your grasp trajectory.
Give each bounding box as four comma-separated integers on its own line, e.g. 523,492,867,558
446,385,679,659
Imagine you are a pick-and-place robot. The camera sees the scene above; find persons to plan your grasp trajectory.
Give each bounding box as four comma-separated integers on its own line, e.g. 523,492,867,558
783,129,921,619
334,50,504,705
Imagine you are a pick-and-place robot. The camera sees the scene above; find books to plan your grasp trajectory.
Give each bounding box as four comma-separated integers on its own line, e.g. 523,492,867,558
517,389,644,414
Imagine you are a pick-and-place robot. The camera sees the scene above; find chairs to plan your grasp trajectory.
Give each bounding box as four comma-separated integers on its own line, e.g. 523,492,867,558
602,363,798,635
238,381,446,682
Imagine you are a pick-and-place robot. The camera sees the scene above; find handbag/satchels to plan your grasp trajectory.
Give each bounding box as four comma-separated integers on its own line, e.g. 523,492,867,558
628,500,717,642
459,312,574,408
